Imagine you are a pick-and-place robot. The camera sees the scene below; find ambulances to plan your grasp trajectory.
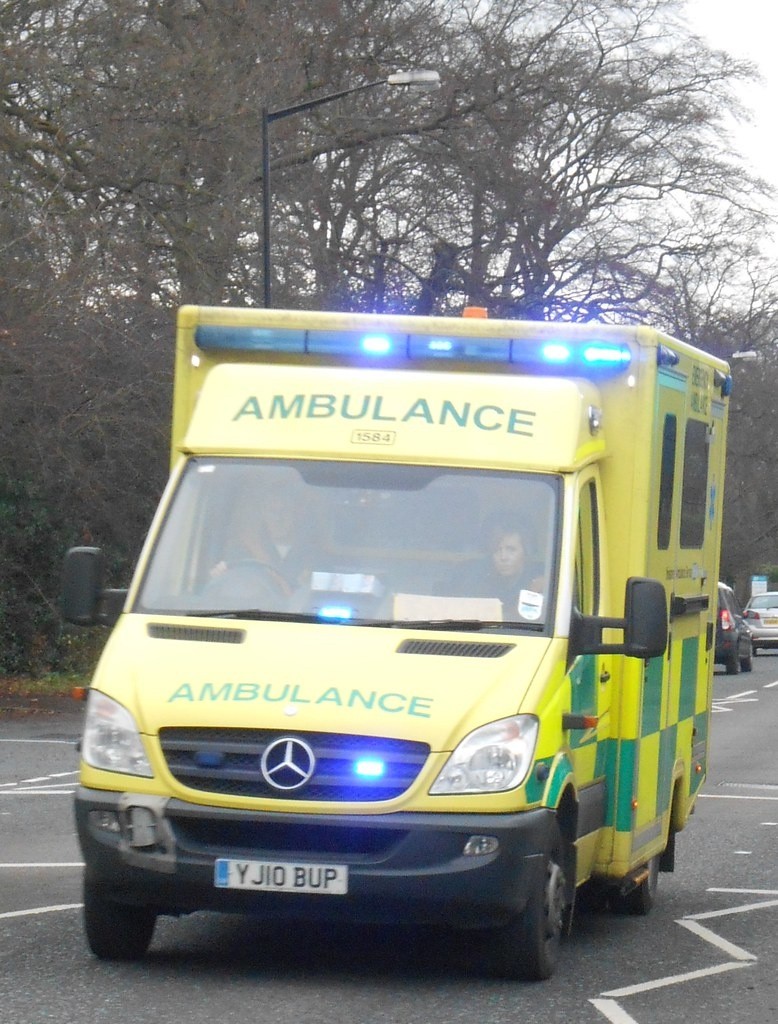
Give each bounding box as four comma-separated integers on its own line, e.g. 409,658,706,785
61,305,732,982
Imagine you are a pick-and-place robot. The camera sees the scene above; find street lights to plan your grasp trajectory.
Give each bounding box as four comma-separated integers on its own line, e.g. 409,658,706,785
263,70,441,308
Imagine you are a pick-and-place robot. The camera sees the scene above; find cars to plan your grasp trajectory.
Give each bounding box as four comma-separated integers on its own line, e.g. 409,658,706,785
715,581,755,675
743,591,778,658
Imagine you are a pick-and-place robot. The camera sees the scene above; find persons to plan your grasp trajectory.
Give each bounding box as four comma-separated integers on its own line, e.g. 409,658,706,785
456,517,547,620
193,487,333,592
